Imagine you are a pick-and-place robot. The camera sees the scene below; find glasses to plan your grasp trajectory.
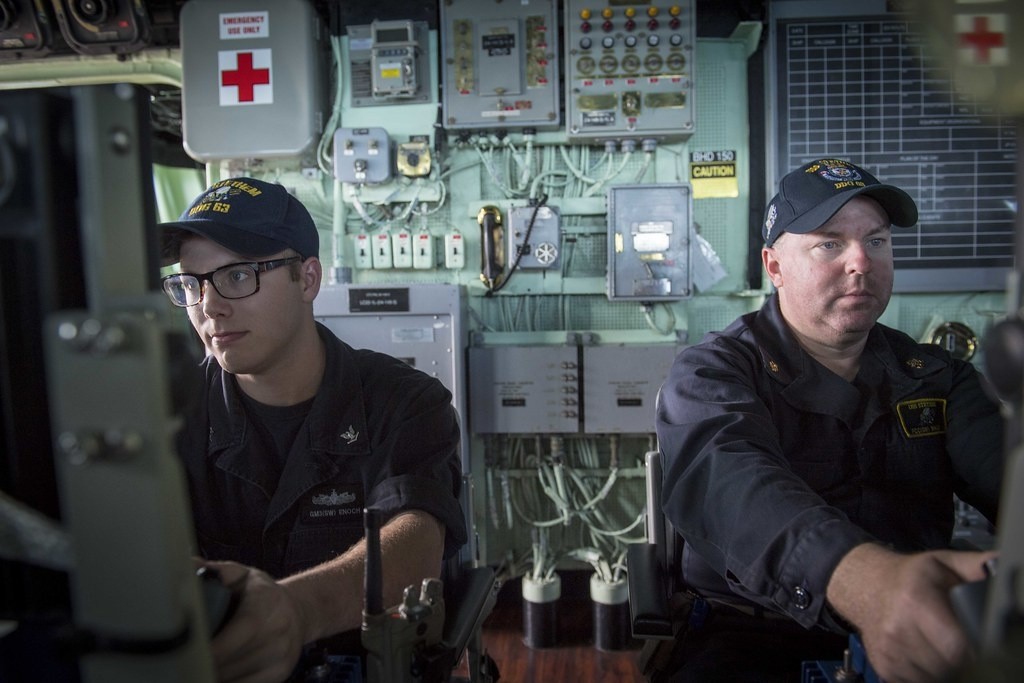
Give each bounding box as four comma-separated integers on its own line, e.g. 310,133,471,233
159,256,304,307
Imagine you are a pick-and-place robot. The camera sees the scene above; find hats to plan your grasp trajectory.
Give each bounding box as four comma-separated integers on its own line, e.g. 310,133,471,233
157,178,320,267
762,158,917,250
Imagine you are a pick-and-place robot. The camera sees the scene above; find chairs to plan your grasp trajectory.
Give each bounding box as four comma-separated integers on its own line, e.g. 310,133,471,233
626,451,697,683
416,404,495,683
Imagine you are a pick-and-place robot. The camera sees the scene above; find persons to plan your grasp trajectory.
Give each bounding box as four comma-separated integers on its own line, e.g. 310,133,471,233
142,178,469,683
650,157,1024,683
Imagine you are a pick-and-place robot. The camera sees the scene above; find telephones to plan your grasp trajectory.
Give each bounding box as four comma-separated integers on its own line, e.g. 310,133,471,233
478,206,505,290
